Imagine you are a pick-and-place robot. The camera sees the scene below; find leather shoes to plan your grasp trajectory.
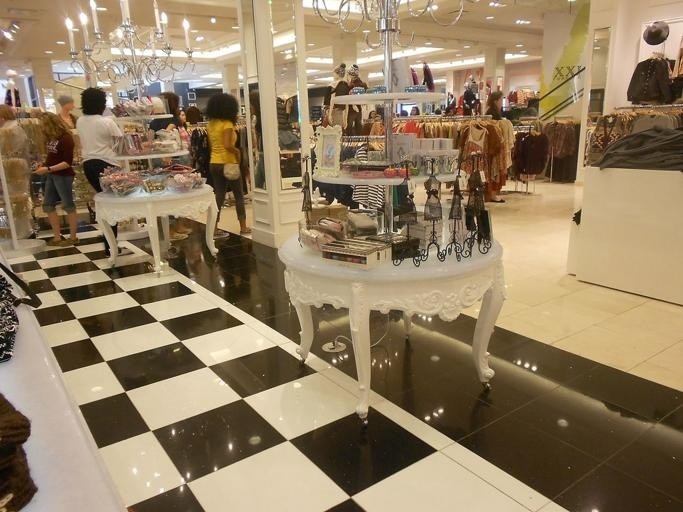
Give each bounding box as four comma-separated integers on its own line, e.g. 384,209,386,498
490,199,505,202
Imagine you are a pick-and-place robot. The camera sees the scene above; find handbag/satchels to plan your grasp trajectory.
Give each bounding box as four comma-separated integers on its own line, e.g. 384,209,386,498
223,163,241,181
1,275,19,362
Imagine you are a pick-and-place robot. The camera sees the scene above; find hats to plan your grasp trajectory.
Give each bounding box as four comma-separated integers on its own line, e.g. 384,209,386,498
643,21,669,45
333,63,346,77
347,65,359,76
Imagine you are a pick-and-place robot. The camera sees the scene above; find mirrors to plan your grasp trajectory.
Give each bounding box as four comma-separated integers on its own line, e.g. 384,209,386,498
270,0,303,190
240,0,265,189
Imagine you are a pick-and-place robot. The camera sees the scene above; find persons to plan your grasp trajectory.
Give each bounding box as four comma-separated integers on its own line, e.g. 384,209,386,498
73,86,126,259
174,88,294,192
147,90,194,244
365,111,376,124
0,103,27,155
410,106,419,116
483,90,506,203
54,93,79,133
345,64,369,136
327,63,350,138
29,110,79,248
203,90,255,239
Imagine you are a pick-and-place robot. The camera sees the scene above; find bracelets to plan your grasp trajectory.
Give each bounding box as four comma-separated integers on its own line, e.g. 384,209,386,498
45,166,53,173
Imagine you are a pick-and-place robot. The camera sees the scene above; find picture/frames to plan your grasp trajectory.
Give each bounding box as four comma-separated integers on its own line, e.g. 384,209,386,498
314,125,342,178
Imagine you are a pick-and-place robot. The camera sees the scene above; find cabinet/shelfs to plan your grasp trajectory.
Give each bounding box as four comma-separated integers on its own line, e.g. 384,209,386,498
277,91,506,420
93,114,220,274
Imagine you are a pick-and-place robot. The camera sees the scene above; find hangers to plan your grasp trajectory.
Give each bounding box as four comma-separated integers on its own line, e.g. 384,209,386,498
629,104,683,117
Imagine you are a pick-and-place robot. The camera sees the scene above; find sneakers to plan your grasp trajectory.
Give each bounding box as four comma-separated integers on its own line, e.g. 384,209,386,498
46,238,79,247
105,248,122,257
213,230,230,239
240,227,251,233
171,229,191,240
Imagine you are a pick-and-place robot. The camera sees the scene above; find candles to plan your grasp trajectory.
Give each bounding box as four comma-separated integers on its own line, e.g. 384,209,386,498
66,0,191,50
